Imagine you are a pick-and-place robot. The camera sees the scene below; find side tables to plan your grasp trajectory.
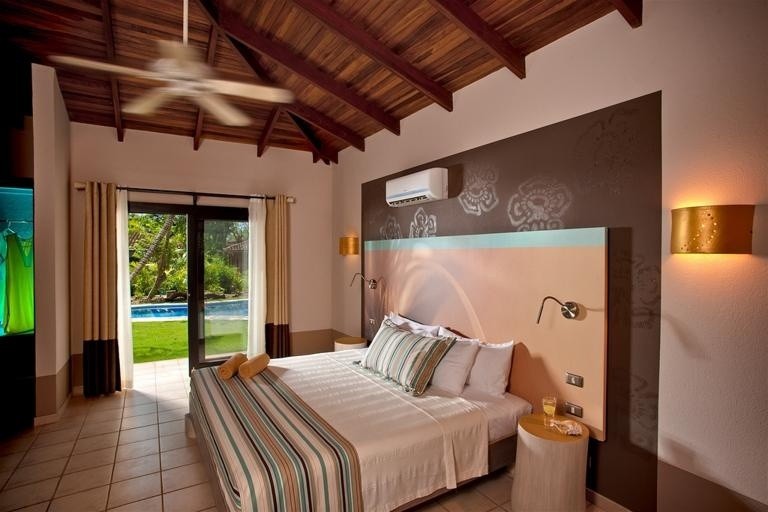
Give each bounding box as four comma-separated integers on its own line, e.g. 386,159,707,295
333,337,367,351
510,413,590,512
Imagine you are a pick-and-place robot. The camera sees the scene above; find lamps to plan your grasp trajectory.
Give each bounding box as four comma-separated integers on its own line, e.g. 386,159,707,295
338,236,359,257
668,202,756,256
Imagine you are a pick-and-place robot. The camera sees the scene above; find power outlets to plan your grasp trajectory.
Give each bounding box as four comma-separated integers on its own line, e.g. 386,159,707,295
564,402,583,418
565,372,584,388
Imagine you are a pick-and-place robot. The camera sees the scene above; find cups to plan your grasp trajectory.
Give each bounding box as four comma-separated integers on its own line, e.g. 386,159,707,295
541,397,556,428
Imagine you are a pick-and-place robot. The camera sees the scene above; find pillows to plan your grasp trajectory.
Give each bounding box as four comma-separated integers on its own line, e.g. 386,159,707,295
389,310,439,339
409,330,478,397
350,315,457,396
437,325,514,400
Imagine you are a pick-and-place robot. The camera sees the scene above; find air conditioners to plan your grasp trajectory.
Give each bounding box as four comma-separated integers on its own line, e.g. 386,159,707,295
385,167,449,208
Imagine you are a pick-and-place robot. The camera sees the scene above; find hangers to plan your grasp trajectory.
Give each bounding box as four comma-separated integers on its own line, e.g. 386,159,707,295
0,219,21,238
3,218,33,247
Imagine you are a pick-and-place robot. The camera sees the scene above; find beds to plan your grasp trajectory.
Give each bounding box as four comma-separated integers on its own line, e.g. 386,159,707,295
184,348,533,512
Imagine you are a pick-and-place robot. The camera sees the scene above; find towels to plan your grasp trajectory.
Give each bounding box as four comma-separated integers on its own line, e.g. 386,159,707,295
217,352,247,380
239,352,270,379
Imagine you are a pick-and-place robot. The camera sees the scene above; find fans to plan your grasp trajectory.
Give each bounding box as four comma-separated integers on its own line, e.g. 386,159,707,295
42,0,295,127
